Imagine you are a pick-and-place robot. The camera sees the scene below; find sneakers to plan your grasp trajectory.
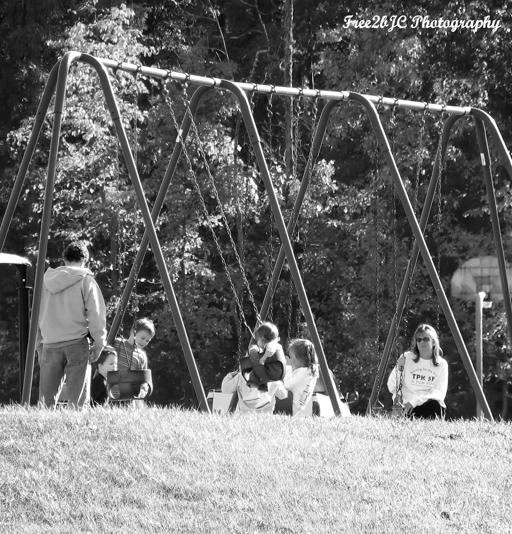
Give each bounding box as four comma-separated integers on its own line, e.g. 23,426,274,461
243,391,270,409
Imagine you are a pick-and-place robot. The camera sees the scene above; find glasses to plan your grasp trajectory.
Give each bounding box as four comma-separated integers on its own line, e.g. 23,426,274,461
416,337,430,342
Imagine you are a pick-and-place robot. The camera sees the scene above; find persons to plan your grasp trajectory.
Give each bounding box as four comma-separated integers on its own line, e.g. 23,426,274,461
90,347,118,405
112,317,157,408
243,320,287,407
38,242,108,408
386,322,448,420
285,337,320,418
220,366,288,414
46,255,95,406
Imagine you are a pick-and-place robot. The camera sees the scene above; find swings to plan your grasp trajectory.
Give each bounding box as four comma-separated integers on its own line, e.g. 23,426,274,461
160,80,269,393
275,97,321,419
394,108,445,416
368,102,404,417
107,66,151,401
